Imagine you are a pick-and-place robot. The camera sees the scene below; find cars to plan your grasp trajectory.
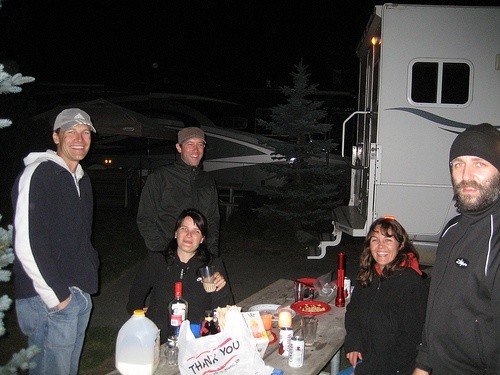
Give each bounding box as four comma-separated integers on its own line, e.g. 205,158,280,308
32,95,351,223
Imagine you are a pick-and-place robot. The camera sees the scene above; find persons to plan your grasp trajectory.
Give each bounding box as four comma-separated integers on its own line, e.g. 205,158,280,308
125,208,236,349
9,106,99,375
343,214,432,375
410,122,500,375
136,126,220,262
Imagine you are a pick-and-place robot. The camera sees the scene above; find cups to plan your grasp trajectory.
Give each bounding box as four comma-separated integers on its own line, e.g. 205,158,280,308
294,282,305,302
199,265,218,293
300,317,318,350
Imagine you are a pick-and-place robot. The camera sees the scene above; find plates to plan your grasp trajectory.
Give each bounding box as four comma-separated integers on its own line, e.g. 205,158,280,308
248,303,300,320
291,300,332,315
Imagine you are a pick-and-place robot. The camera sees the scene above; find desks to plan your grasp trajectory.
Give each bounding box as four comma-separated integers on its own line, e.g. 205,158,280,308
217,184,241,218
106,279,347,375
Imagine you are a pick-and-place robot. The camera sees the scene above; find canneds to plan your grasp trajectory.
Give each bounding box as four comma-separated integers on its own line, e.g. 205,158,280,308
279,327,293,357
288,336,305,367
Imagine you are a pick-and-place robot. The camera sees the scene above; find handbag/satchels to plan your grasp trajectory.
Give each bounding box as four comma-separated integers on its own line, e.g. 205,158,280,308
177,304,274,375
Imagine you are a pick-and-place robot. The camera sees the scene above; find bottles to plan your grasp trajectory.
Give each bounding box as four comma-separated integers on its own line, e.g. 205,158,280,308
202,309,219,337
166,282,188,351
164,342,178,368
114,310,161,375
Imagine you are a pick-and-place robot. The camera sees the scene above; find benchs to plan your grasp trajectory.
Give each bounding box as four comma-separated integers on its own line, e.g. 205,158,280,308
219,203,240,222
219,193,244,208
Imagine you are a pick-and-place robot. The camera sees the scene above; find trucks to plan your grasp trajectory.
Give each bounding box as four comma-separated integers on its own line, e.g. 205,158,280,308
330,3,500,278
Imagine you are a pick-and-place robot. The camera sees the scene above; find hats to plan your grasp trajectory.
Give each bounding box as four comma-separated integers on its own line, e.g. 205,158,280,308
449,122,500,172
54,108,96,133
177,127,206,148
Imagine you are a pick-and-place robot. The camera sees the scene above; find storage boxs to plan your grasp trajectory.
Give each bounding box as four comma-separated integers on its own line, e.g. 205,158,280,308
241,310,270,359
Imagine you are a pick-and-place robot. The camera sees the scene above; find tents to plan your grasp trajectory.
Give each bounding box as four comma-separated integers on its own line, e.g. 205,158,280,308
33,97,180,197
